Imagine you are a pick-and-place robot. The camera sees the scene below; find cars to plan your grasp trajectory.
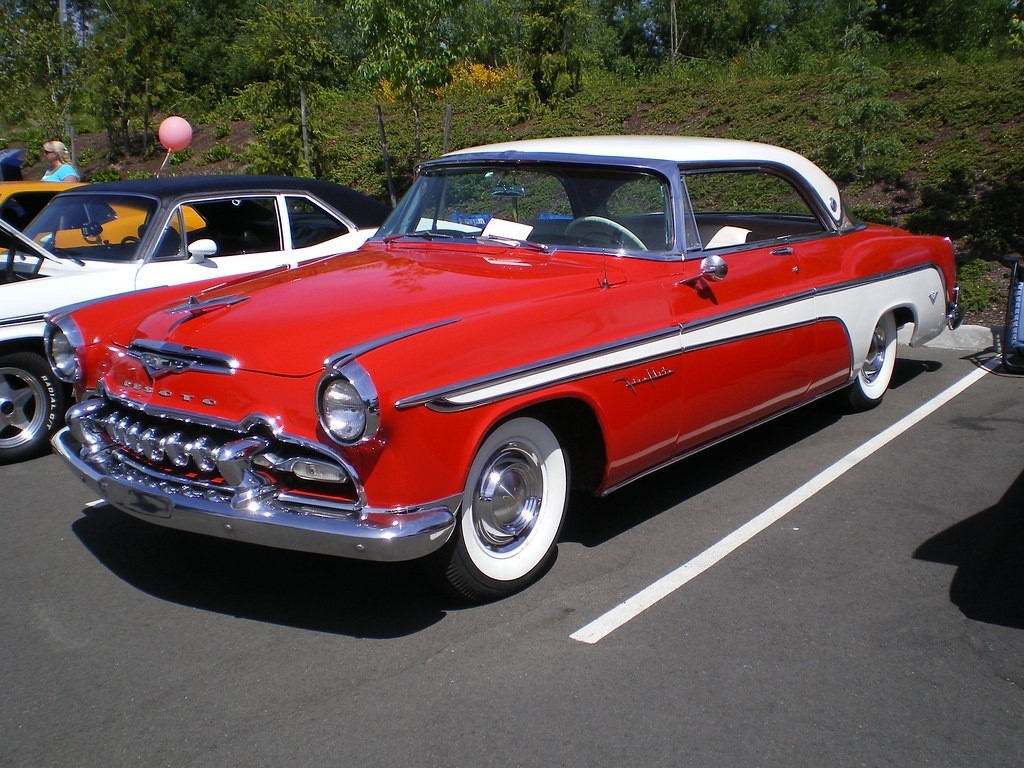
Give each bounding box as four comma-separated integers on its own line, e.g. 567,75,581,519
0,180,207,255
0,174,482,469
39,132,965,608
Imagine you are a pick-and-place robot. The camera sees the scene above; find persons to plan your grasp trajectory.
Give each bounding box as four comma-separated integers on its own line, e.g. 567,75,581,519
41,141,77,183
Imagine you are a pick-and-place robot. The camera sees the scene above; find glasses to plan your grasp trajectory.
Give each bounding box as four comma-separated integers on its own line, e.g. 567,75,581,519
44,150,52,154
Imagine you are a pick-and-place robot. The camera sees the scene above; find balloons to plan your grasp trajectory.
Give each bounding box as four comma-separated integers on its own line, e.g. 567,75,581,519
159,116,192,151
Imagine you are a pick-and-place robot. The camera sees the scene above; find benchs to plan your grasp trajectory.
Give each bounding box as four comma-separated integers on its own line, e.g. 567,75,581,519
208,220,342,252
524,214,821,253
